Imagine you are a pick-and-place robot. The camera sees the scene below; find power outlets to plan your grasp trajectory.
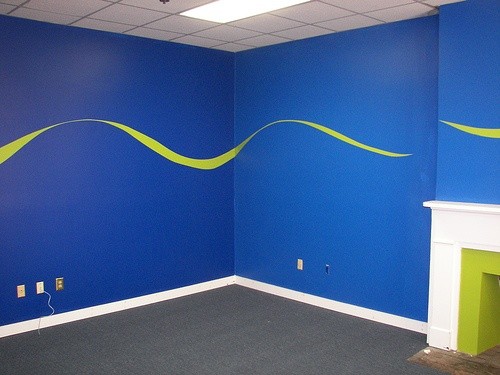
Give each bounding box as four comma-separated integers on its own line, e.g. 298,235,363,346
17,285,25,298
36,281,44,294
